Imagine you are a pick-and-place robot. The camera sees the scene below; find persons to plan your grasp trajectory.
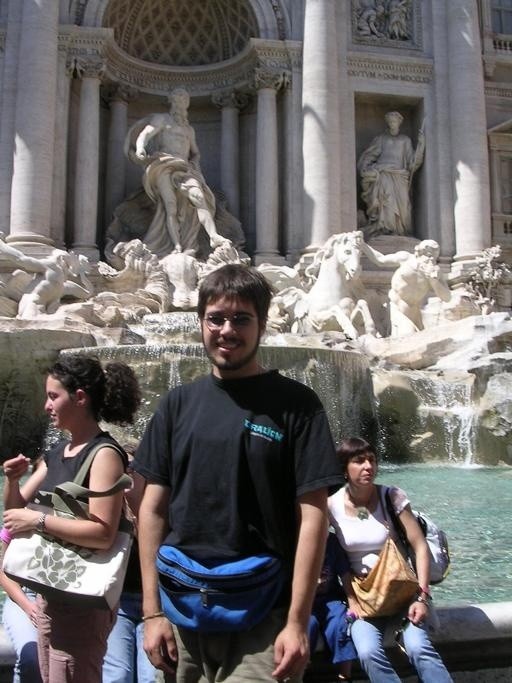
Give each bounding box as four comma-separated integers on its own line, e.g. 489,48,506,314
357,112,425,234
389,0,409,41
130,264,346,683
352,231,451,336
358,6,387,37
309,437,455,683
135,84,236,254
0,239,94,318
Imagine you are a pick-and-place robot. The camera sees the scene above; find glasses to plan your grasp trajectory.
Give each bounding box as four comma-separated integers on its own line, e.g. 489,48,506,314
201,314,261,329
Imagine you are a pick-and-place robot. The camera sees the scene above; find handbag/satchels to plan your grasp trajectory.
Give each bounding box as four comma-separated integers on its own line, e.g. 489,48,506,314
157,544,282,634
2,442,135,611
342,484,452,619
